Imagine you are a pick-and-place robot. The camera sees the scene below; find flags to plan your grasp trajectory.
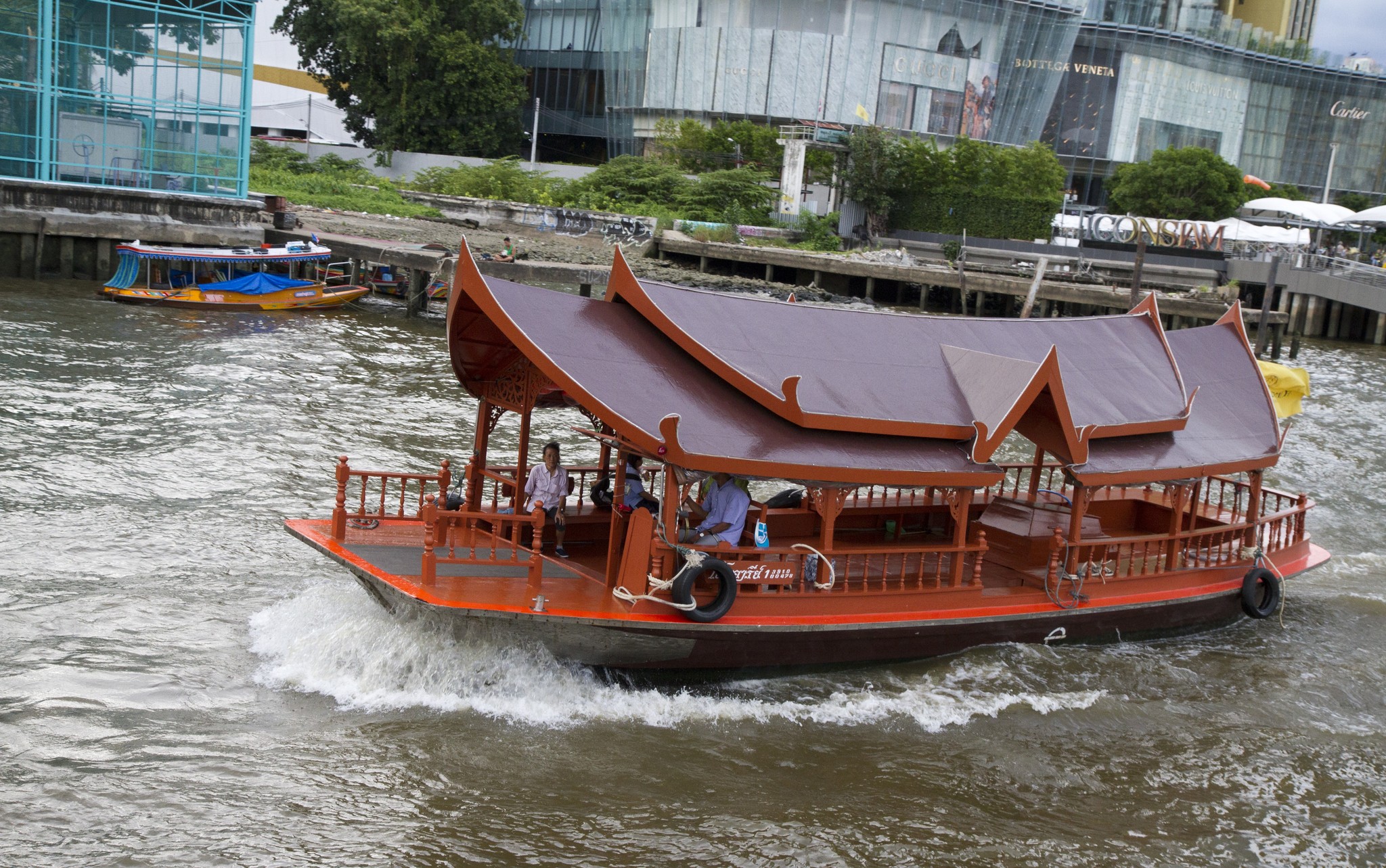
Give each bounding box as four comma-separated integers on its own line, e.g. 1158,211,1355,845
311,232,320,243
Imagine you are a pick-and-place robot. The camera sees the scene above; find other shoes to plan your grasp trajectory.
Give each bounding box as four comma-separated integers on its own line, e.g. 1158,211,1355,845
540,543,544,553
555,548,569,557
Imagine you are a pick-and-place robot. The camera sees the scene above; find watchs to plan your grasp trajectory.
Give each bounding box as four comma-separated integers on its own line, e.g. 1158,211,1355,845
524,441,571,557
699,532,704,538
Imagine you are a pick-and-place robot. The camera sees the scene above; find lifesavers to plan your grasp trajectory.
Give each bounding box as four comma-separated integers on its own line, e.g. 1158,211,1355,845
1241,568,1279,619
671,556,737,624
395,281,406,292
432,493,466,512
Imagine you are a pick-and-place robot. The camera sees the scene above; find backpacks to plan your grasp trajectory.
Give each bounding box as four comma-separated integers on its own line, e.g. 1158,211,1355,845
589,473,642,507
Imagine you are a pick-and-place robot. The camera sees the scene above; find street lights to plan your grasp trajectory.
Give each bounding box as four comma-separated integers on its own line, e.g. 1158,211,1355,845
1319,140,1342,208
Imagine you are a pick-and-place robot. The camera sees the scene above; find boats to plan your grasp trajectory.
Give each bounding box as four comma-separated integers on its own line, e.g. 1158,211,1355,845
96,240,372,314
283,234,1338,680
280,257,453,302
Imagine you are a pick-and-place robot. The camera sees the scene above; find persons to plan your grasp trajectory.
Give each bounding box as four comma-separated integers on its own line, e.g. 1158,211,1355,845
1309,238,1350,269
494,237,516,263
678,471,750,563
700,472,752,512
622,453,664,518
1370,247,1383,270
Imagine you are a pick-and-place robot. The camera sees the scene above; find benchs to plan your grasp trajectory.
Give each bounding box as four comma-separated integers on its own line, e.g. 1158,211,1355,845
1016,533,1289,589
480,485,1149,548
691,490,770,593
1133,484,1288,547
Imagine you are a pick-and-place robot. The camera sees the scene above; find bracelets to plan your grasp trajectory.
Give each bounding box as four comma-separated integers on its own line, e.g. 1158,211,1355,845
704,496,706,500
